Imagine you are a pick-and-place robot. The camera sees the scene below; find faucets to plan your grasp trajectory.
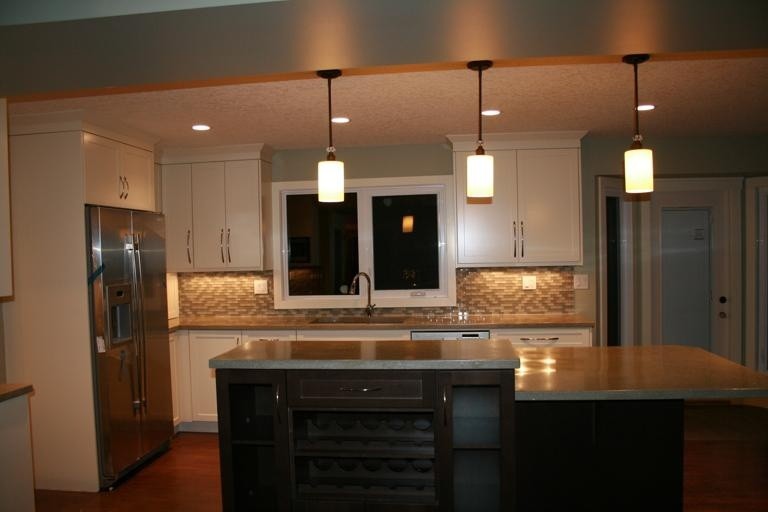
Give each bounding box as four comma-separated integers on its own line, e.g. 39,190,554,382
350,271,376,315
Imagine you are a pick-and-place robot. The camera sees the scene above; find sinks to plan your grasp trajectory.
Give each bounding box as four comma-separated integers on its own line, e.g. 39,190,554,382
311,317,405,323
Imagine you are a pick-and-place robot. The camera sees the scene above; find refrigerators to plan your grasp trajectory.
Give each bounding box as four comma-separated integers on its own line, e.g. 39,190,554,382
85,203,176,489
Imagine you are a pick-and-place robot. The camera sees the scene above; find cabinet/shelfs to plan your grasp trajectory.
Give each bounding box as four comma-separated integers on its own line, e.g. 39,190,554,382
193,144,274,272
190,329,241,432
11,131,155,211
492,328,590,346
160,148,193,272
453,131,582,268
